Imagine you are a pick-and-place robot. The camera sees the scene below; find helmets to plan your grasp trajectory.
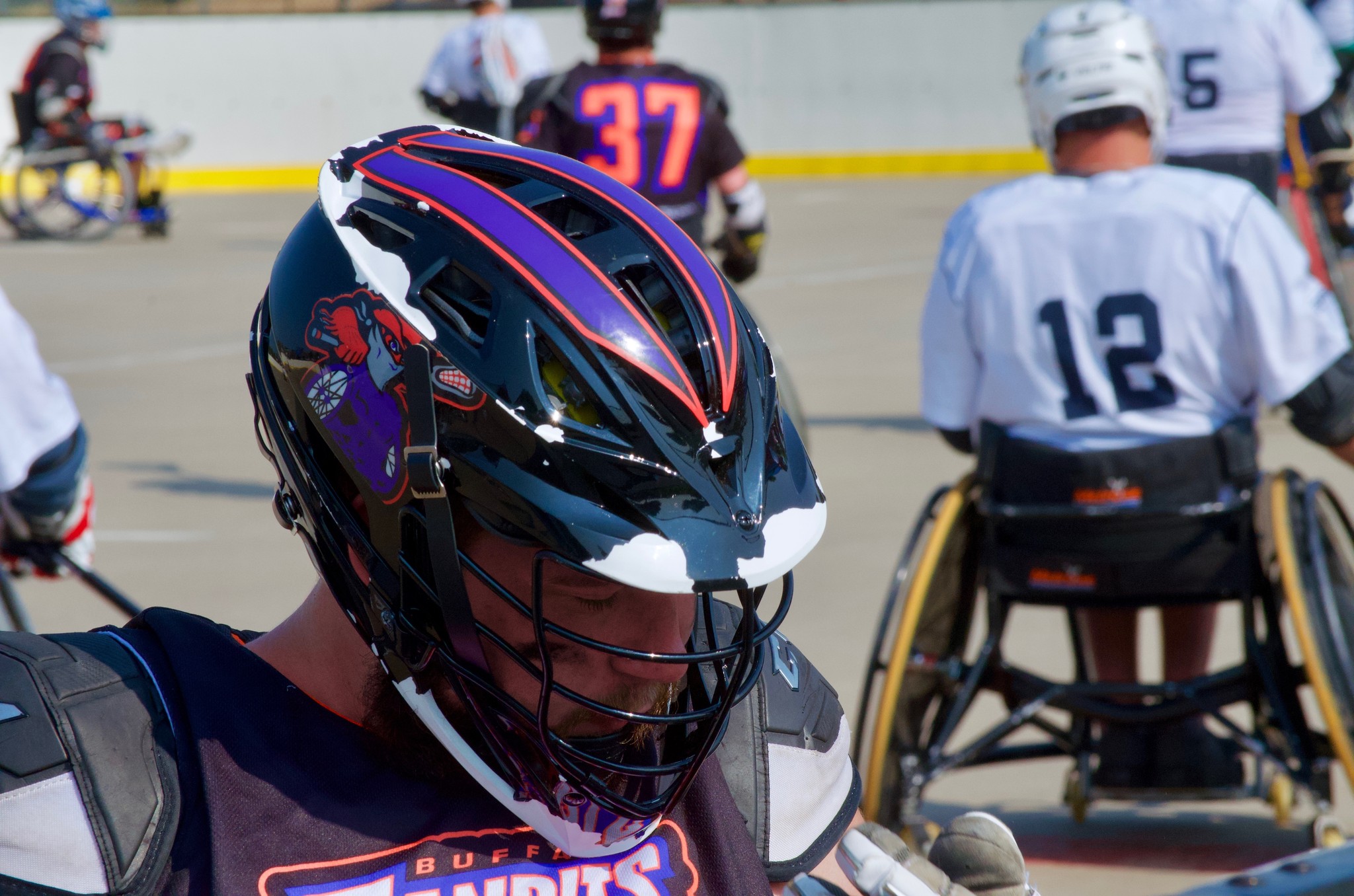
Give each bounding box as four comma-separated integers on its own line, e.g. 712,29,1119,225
53,0,112,50
246,124,834,865
1020,1,1169,162
583,0,660,48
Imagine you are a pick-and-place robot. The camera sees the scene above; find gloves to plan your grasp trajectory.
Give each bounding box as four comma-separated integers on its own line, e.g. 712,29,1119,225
780,812,1042,896
81,120,114,169
0,473,96,580
1318,173,1354,248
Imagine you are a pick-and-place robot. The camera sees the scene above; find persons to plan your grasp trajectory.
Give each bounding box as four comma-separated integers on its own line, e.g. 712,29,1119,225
1,290,96,634
19,1,161,216
510,1,766,285
1,124,1033,895
418,0,555,140
920,1,1354,791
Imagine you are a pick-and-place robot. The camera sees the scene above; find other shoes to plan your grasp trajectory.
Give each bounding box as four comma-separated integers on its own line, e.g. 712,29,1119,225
1091,715,1251,803
136,191,167,237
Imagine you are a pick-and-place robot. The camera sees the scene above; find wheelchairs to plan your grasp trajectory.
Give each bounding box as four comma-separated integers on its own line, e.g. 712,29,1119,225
0,92,190,242
846,449,1354,851
